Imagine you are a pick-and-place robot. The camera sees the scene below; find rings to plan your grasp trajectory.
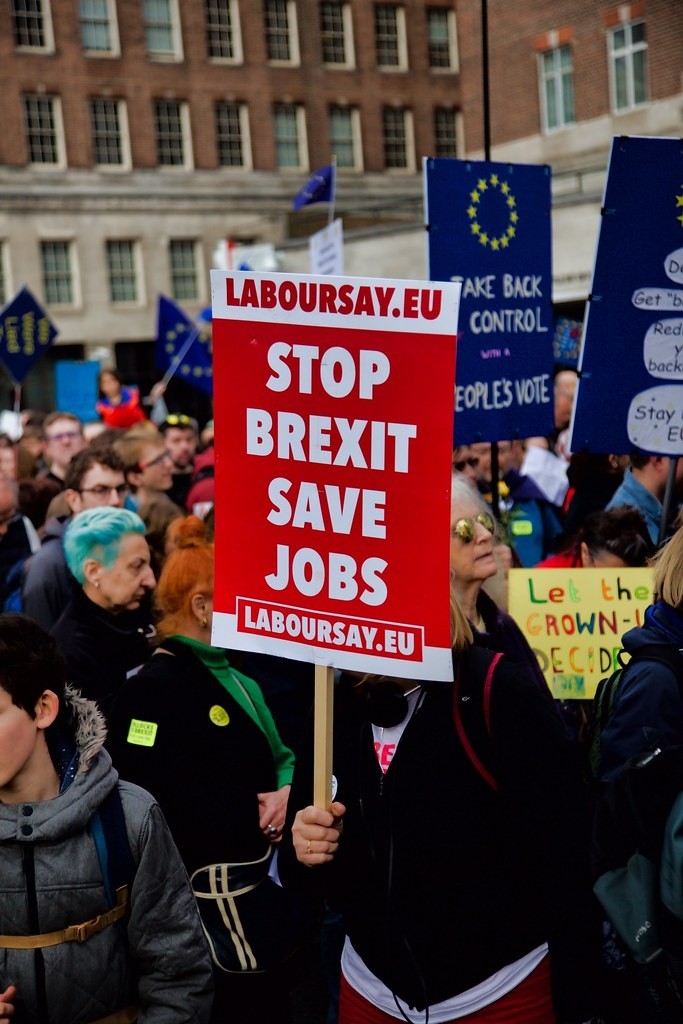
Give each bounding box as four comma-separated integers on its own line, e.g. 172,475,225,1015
270,827,277,834
306,841,312,854
268,824,271,828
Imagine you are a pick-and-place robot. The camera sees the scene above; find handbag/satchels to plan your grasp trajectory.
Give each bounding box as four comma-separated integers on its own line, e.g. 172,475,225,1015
190,845,275,974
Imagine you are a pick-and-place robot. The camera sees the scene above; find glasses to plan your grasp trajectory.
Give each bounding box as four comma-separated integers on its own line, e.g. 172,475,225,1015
455,457,479,471
135,449,174,472
166,414,192,426
47,432,82,442
81,483,129,498
451,512,494,543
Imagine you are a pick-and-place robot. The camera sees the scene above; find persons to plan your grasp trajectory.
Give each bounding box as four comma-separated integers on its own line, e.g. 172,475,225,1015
0,365,683,1024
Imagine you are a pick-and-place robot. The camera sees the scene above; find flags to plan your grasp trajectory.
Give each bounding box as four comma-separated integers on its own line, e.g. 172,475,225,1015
159,300,213,395
294,164,334,214
